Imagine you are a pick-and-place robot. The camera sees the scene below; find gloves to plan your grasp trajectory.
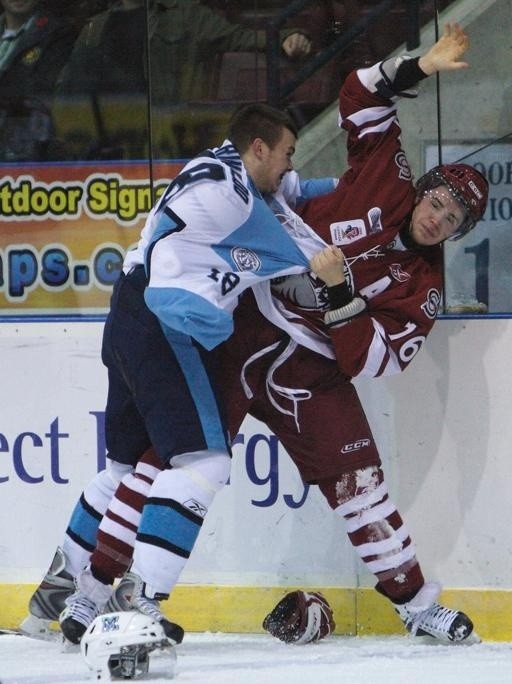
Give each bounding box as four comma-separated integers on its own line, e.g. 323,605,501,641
262,590,337,645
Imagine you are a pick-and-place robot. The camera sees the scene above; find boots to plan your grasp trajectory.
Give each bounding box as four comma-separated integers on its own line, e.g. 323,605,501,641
58,565,115,644
375,578,473,641
105,558,186,645
27,543,77,621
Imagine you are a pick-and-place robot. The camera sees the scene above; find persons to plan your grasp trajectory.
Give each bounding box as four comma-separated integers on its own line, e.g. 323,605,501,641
61,12,492,647
25,96,344,645
45,1,318,162
1,0,78,162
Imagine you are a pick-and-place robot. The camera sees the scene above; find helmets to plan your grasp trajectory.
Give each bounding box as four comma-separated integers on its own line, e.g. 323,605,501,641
80,610,167,681
415,162,489,243
